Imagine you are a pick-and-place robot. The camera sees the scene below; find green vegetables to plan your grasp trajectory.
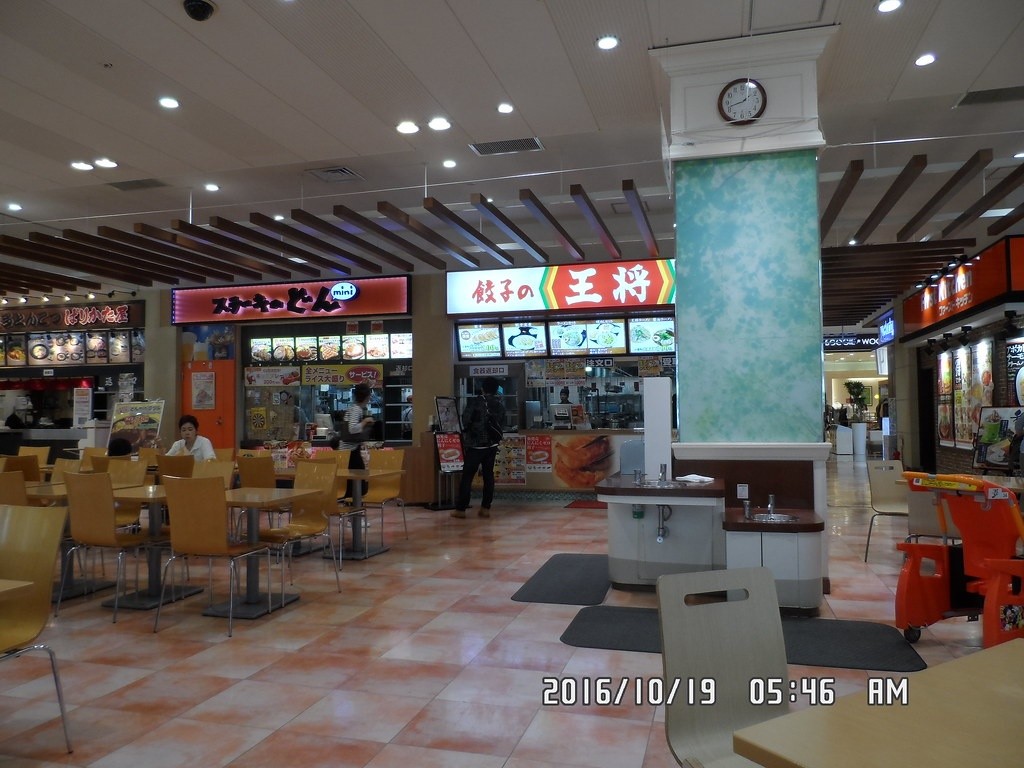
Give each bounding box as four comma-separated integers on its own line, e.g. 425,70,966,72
654,332,671,345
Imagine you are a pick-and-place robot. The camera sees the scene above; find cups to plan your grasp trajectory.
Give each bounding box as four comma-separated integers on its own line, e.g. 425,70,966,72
981,421,1000,443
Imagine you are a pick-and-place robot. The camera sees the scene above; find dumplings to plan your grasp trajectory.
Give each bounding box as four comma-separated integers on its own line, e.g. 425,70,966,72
472,331,498,344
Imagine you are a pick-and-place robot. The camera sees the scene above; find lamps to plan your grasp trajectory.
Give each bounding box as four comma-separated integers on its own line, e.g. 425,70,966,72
86,329,93,339
43,294,69,301
85,291,112,299
46,331,51,340
924,339,937,356
64,293,87,300
948,258,957,267
67,330,72,339
132,327,138,337
6,331,31,342
1,297,19,304
958,325,972,346
107,290,137,298
915,281,923,288
931,271,939,278
110,329,115,338
1003,310,1017,333
20,296,43,302
940,333,952,351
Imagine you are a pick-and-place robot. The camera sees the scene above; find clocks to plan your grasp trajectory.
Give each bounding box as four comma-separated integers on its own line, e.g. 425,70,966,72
718,77,767,125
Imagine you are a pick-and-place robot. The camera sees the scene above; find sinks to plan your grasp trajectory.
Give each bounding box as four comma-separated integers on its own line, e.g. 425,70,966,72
640,481,687,488
752,513,800,522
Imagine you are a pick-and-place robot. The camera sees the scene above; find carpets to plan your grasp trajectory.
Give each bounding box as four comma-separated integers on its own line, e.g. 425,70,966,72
559,605,927,673
510,553,611,605
563,499,608,509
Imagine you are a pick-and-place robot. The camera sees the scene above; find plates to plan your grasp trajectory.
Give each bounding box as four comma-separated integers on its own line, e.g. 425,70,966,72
986,455,1009,466
6,346,26,361
508,323,674,351
251,339,366,363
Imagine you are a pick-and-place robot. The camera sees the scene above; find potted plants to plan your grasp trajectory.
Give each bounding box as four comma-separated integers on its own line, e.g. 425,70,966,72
844,381,868,454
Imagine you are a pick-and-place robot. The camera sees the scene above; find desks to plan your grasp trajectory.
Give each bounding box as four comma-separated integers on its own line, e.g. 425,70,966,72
201,488,325,620
321,468,406,560
733,637,1024,768
24,482,142,605
894,476,1024,493
101,486,204,611
254,468,331,559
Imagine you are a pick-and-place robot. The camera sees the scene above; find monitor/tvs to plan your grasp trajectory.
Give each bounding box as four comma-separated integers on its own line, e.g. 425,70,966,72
550,404,572,423
315,414,334,431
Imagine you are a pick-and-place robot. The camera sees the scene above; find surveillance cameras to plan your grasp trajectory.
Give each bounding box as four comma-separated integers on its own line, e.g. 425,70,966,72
183,0,217,22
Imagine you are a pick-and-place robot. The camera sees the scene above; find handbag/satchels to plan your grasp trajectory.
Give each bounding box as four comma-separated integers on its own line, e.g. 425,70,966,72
481,394,504,443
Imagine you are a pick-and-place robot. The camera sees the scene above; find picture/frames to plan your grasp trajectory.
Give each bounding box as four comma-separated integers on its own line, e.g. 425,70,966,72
860,386,873,406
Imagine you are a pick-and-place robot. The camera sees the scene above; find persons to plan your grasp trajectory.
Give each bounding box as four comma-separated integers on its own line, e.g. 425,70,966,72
559,389,572,404
165,414,216,460
338,384,375,527
402,395,413,440
450,376,504,518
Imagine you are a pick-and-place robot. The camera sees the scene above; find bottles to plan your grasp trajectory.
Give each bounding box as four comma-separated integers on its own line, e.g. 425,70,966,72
743,501,751,519
768,494,774,514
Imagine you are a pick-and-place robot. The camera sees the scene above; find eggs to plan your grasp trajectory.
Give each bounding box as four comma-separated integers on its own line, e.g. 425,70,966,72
653,336,660,341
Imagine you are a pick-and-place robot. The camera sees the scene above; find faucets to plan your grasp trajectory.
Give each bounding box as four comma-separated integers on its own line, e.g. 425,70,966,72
658,464,667,481
634,469,648,482
767,495,775,514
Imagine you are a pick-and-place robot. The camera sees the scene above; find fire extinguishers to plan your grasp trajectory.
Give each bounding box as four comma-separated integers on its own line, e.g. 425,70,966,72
892,447,900,460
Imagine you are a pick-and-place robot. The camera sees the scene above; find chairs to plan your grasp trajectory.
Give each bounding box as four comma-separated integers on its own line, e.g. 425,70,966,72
0,445,1024,768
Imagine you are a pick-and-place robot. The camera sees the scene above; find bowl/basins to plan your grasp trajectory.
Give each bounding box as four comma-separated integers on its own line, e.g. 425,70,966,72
31,338,142,360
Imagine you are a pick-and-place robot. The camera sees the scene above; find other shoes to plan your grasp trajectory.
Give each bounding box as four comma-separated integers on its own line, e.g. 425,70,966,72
346,517,371,528
450,509,465,518
478,508,490,517
